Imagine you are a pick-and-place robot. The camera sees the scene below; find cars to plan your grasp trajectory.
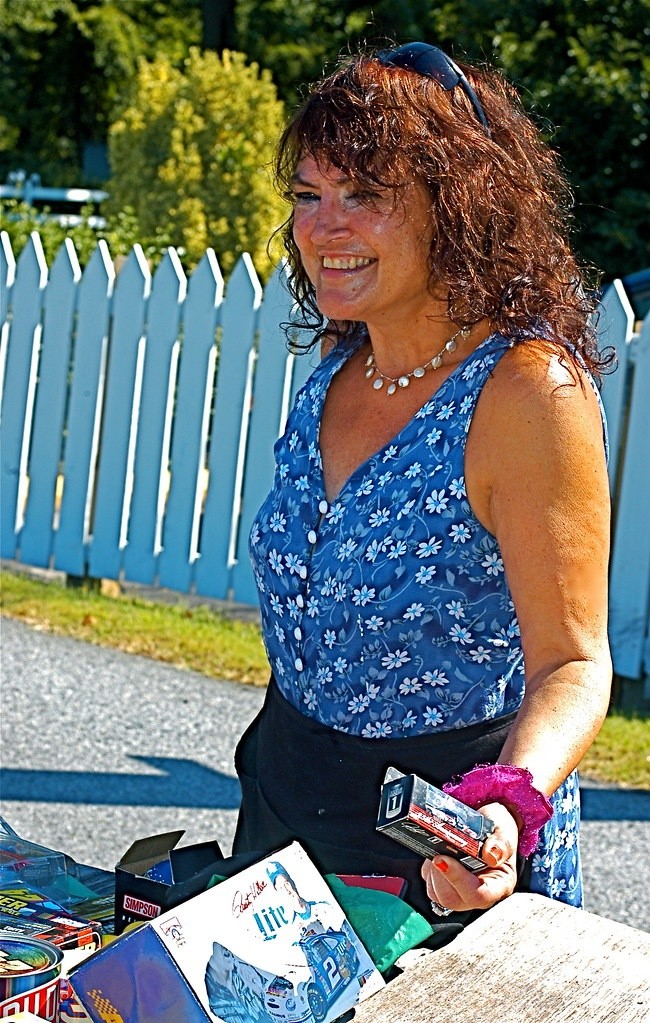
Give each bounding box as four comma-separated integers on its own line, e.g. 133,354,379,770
261,930,359,1023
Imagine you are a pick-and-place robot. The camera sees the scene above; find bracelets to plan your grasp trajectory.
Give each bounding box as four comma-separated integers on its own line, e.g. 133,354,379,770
442,762,554,858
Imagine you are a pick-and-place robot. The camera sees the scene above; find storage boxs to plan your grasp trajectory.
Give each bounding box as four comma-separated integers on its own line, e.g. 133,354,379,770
114,829,225,938
62,840,387,1023
0,830,69,901
0,893,104,952
375,766,496,875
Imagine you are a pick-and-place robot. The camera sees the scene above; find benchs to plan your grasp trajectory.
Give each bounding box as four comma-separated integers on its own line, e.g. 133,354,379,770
14,214,107,230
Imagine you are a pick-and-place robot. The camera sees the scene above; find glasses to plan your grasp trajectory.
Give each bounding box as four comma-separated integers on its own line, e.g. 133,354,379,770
369,41,491,140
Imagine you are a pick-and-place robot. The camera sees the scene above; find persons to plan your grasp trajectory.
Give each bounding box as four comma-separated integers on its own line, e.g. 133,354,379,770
231,41,613,945
266,861,342,982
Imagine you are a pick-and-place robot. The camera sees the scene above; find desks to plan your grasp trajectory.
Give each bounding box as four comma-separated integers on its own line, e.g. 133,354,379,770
0,186,108,216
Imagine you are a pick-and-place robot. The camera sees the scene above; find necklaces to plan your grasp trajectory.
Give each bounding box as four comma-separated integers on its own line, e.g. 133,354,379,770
364,325,471,397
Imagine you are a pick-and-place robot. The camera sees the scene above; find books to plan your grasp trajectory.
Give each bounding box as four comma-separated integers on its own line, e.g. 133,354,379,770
67,842,387,1023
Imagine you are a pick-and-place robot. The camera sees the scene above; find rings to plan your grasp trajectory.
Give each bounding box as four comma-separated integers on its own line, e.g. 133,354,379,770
431,901,454,917
